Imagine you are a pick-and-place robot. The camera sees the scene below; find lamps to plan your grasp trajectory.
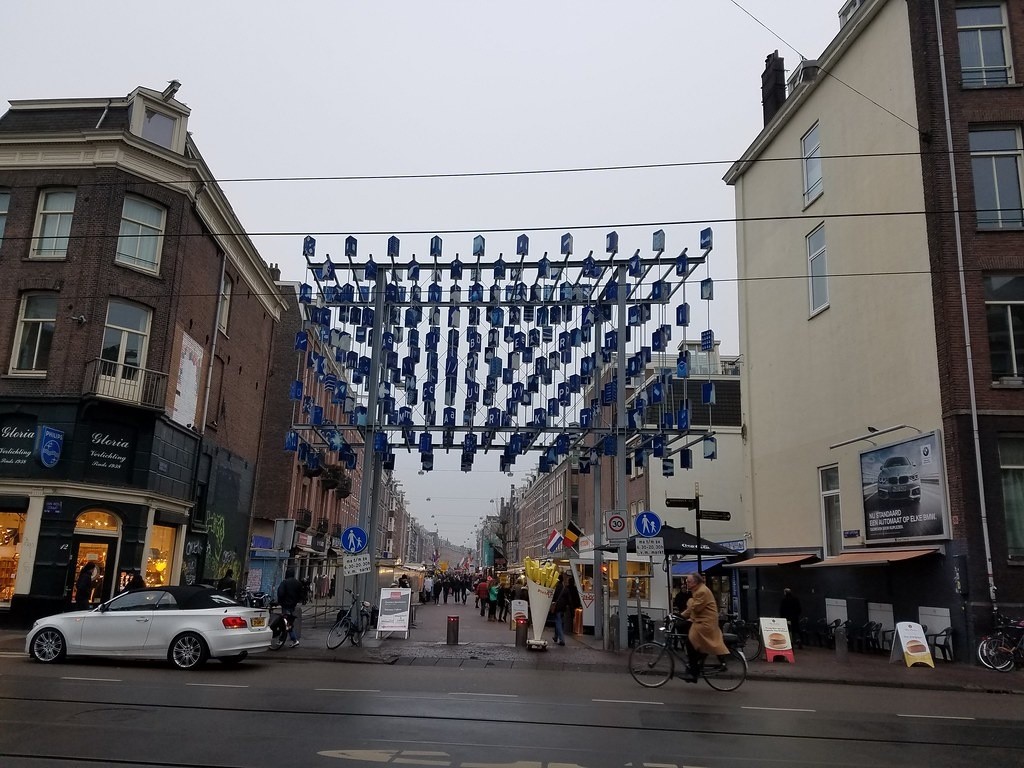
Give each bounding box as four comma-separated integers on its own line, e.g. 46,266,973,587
69,315,86,327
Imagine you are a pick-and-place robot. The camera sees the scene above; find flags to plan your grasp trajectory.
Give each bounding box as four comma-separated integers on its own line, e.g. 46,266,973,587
459,551,472,568
562,520,582,548
544,529,563,553
429,548,440,565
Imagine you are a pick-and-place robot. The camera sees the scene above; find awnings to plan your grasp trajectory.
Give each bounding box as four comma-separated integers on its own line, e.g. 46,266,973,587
800,550,937,568
327,548,347,557
670,560,725,576
722,555,815,566
294,545,321,554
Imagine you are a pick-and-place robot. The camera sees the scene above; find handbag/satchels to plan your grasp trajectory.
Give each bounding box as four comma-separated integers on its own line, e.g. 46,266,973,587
551,602,556,613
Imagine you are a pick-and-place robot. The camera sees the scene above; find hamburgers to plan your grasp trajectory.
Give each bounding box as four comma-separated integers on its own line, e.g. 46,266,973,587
768,633,788,648
906,640,926,654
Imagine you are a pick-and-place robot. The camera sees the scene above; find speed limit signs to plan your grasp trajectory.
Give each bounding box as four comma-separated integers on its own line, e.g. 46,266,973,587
609,516,625,531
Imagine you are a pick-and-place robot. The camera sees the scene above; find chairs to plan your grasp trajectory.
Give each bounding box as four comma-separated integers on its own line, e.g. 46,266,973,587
798,616,955,665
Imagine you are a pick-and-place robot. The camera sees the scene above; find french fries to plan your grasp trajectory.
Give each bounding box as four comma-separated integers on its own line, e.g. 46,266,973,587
523,556,560,589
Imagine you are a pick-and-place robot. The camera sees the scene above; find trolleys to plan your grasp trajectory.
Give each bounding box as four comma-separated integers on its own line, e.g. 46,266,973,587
525,639,548,652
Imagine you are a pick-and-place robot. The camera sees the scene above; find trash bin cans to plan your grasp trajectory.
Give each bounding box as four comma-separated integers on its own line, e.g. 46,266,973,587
629,612,654,650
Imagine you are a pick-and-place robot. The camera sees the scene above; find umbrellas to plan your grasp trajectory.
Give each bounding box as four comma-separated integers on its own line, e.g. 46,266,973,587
592,521,741,613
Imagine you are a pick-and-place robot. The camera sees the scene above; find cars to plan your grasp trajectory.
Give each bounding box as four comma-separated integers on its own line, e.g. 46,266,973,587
23,586,274,671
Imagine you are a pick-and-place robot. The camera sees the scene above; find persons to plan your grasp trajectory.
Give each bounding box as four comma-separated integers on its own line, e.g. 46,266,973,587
423,568,533,623
216,569,236,601
268,614,295,649
398,575,412,588
552,576,566,645
669,573,730,683
629,579,646,599
390,583,396,588
563,578,581,633
276,568,302,647
119,569,146,593
75,562,98,611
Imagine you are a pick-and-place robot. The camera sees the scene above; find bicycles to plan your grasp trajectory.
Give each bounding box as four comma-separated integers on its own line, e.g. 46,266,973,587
628,615,748,691
303,587,313,606
718,615,762,662
976,614,1024,672
325,588,373,650
222,588,294,651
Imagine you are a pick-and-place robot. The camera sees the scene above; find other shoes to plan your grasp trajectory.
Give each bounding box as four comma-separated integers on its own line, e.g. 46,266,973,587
558,641,565,645
679,673,699,684
289,640,299,648
276,641,282,645
475,606,479,608
435,599,466,607
488,616,506,623
552,636,558,642
